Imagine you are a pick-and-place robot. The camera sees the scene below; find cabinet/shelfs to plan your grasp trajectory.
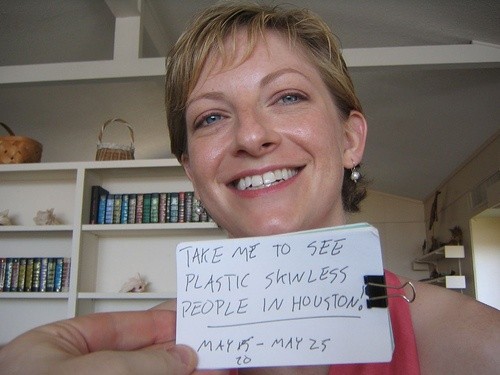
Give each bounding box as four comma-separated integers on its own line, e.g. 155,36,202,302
411,238,464,297
0,159,227,347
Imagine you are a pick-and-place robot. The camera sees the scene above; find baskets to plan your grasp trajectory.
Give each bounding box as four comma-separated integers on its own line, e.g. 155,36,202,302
96,116,135,161
0,122,43,163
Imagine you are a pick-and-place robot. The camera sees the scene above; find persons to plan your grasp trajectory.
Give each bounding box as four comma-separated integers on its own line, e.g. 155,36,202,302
0,310,198,375
129,1,500,375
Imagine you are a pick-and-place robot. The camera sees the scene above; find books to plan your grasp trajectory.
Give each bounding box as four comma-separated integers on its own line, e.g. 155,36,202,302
90,186,214,224
0,257,71,292
